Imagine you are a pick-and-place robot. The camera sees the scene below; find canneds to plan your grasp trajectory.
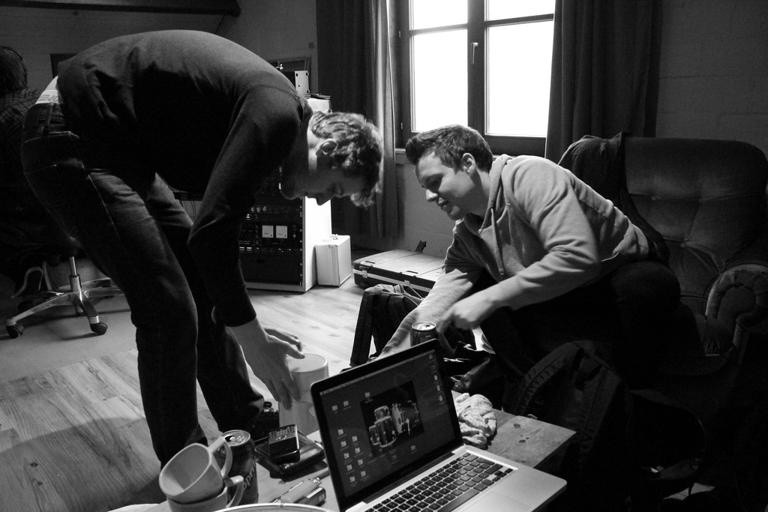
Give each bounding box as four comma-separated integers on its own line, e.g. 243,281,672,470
214,428,260,507
411,320,440,351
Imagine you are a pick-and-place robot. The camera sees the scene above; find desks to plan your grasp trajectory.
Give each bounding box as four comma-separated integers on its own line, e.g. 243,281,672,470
114,388,576,511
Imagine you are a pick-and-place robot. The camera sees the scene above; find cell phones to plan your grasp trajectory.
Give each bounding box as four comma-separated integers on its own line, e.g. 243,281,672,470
267,423,300,463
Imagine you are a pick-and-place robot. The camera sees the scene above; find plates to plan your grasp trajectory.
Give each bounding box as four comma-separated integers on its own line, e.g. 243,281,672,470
212,503,331,512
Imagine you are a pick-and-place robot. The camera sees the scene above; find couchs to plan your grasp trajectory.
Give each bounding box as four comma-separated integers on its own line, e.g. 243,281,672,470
545,131,767,512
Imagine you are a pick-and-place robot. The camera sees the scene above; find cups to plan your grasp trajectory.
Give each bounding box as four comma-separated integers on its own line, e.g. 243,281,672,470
279,352,330,435
158,438,244,512
368,406,396,448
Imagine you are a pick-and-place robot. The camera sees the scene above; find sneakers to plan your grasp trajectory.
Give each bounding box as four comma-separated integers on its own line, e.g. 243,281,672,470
256,401,279,430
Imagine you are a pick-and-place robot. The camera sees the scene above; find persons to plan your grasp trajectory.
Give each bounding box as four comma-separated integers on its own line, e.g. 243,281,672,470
372,123,682,512
23,28,385,465
0,44,42,298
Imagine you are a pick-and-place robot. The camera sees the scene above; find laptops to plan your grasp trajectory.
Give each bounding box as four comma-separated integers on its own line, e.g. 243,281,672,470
307,339,567,512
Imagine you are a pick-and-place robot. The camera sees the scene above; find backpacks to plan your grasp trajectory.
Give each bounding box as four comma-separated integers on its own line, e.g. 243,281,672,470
351,276,470,386
505,339,636,512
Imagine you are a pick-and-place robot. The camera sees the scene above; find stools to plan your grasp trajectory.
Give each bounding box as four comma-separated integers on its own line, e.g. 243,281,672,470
1,224,126,339
